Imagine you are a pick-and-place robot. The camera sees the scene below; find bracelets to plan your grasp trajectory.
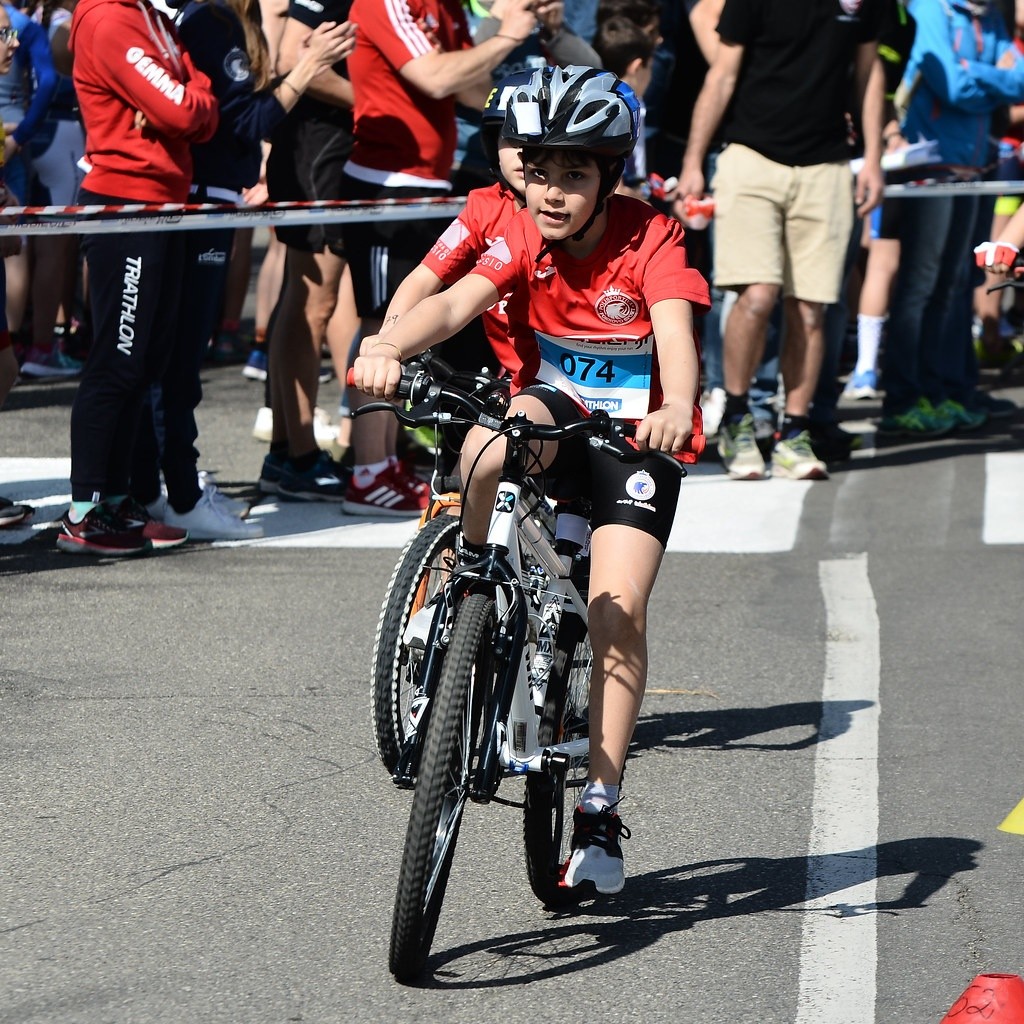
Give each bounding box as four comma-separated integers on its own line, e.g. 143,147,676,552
283,79,302,99
371,342,402,363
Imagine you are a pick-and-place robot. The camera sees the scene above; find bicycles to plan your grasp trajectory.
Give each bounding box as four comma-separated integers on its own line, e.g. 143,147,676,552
370,350,596,775
344,366,706,976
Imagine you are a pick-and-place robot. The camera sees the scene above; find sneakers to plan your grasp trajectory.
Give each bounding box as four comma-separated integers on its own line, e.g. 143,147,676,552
767,429,829,482
843,370,880,400
56,498,144,554
197,471,256,522
974,337,1024,367
276,452,351,501
260,451,294,494
20,343,82,377
0,498,36,526
343,463,433,516
970,313,1016,339
934,397,984,430
405,592,445,648
162,496,266,540
106,499,190,550
147,493,169,519
717,416,764,479
878,399,953,437
967,389,1015,417
564,801,631,895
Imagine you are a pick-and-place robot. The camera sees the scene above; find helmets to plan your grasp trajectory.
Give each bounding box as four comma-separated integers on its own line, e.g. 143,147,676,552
483,69,531,174
499,65,643,154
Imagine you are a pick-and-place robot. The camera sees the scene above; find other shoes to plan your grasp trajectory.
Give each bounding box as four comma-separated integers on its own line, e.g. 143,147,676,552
749,402,777,441
209,330,244,364
699,387,727,439
245,351,271,384
813,418,862,447
255,404,342,446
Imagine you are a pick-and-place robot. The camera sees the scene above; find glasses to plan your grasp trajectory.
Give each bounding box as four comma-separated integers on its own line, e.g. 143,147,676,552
0,28,17,44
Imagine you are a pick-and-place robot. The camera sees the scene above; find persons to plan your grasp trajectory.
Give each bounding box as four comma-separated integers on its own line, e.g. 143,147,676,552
676,0,885,478
357,76,525,524
352,65,711,897
0,0,1024,556
